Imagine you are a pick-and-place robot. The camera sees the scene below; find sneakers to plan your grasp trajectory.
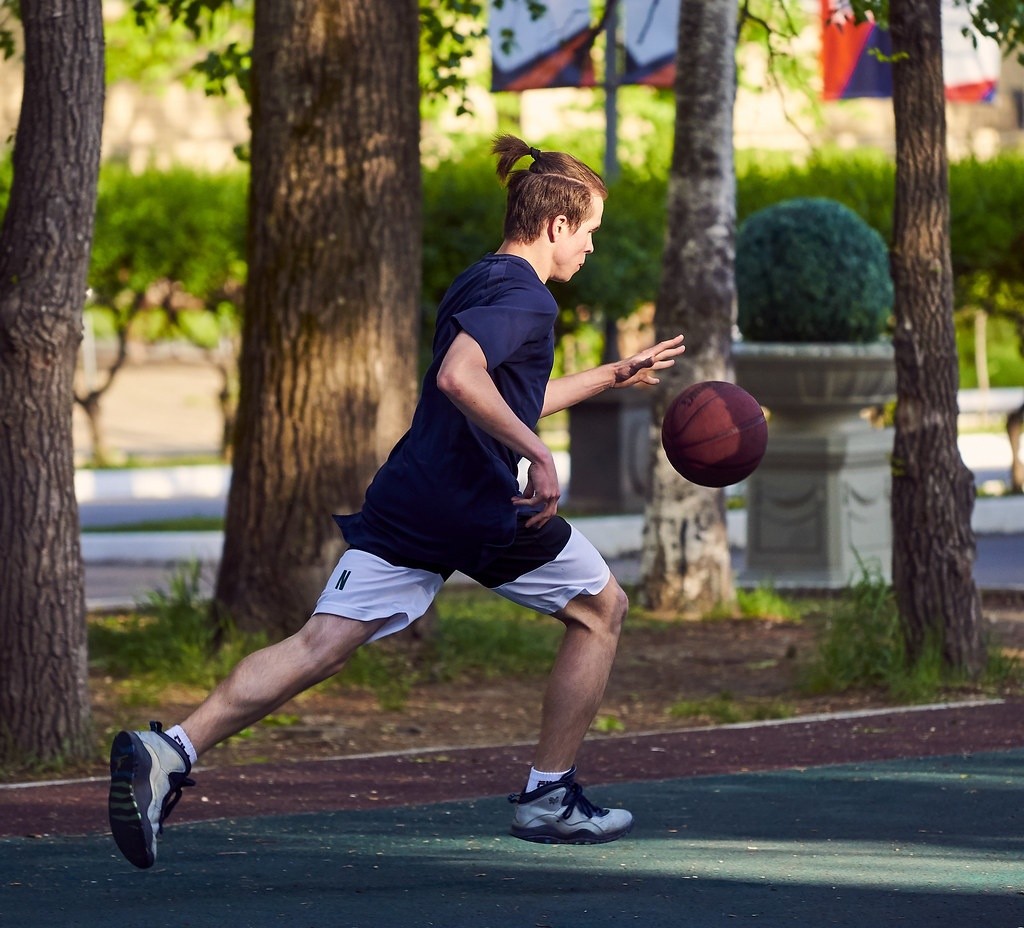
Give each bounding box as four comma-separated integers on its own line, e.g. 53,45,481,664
507,763,634,844
108,718,198,869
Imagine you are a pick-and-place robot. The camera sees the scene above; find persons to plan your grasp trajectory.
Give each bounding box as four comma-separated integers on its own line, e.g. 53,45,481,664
106,136,691,869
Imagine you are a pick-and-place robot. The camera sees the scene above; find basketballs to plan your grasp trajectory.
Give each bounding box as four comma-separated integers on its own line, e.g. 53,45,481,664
661,379,770,489
